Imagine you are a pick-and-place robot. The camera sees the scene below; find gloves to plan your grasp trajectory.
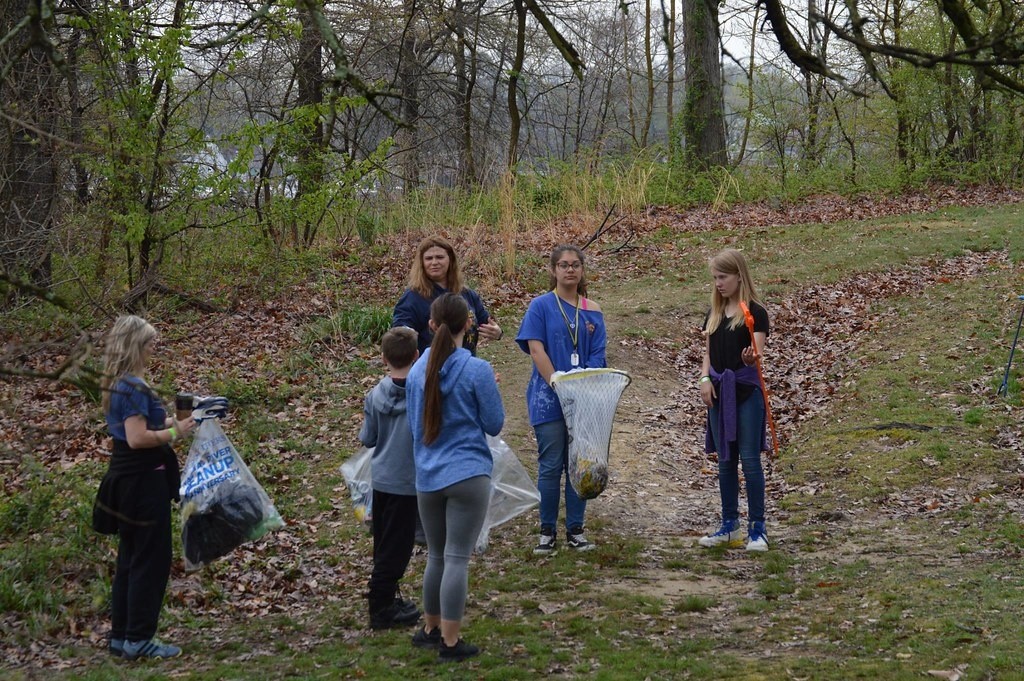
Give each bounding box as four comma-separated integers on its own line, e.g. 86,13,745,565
192,396,229,422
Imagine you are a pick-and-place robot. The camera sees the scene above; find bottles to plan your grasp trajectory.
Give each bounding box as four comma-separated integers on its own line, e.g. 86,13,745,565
339,465,373,533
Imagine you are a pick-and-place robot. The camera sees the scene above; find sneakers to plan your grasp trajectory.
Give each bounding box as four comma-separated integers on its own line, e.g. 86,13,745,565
746,520,768,553
699,519,742,547
412,624,442,650
438,636,478,664
395,598,417,607
566,525,596,552
109,638,125,657
376,607,421,627
123,639,183,660
533,526,557,554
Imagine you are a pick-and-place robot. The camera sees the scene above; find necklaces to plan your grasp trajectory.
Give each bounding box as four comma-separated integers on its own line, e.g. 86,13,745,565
562,306,576,328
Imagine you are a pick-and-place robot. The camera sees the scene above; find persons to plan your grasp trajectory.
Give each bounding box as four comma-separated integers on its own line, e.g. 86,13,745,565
407,293,505,659
393,234,504,549
515,246,607,554
698,250,769,551
358,326,420,630
92,314,197,657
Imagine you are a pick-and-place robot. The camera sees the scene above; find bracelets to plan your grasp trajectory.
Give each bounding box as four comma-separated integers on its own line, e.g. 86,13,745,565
168,428,176,442
496,330,504,341
699,376,710,383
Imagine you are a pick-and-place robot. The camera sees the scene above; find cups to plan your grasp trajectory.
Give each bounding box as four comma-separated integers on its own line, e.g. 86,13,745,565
176,391,194,421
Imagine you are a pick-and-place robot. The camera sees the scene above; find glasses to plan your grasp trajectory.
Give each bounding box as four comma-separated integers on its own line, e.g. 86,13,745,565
556,260,582,271
